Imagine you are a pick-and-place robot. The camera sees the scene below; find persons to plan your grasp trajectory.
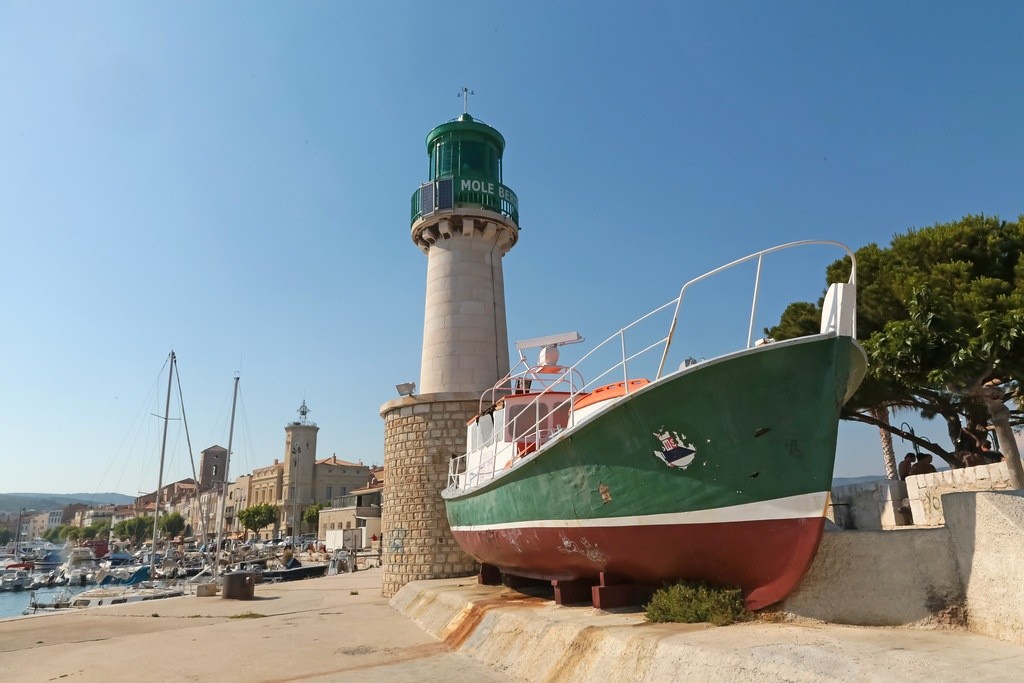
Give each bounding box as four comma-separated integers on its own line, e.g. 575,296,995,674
980,440,1001,463
899,452,938,481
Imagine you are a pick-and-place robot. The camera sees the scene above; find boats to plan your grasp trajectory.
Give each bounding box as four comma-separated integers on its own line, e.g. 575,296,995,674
0,349,329,592
439,238,868,616
